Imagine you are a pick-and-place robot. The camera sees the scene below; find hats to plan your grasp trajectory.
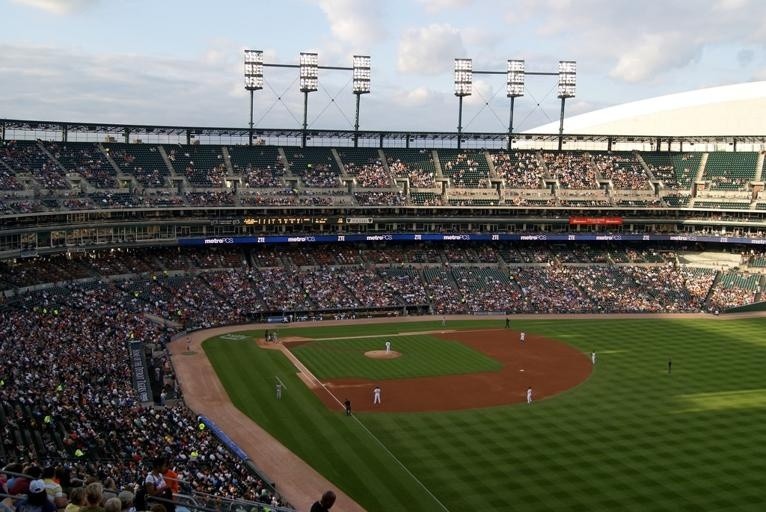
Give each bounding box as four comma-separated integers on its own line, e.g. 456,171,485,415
30,479,46,493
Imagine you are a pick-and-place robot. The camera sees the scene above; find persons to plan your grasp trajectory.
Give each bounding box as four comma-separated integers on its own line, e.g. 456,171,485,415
520,330,525,343
386,340,391,352
344,398,352,416
373,387,381,404
668,358,672,374
0,276,336,512
0,142,766,329
527,386,532,404
591,349,596,364
